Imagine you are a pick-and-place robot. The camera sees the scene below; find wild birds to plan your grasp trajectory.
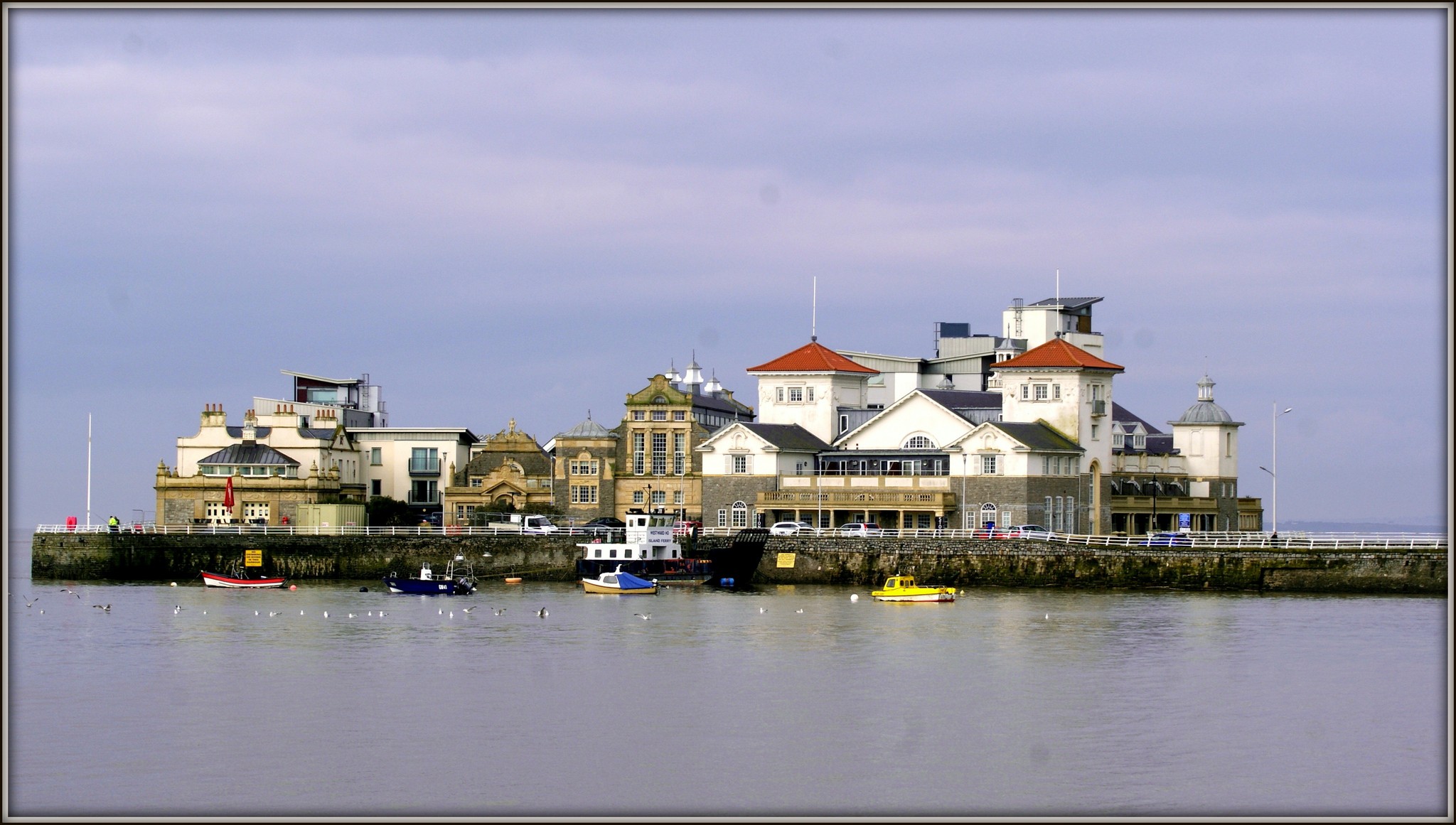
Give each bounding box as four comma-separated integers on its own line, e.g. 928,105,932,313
8,588,113,614
794,609,804,614
634,613,651,620
760,608,768,614
173,605,551,617
1045,614,1048,620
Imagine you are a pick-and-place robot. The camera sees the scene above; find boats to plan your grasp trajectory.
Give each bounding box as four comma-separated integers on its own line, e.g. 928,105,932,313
575,483,772,590
381,547,480,597
582,564,670,595
199,558,292,589
871,570,957,603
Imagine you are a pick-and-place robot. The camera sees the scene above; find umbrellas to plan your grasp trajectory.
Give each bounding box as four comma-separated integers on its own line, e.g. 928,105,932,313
224,476,235,524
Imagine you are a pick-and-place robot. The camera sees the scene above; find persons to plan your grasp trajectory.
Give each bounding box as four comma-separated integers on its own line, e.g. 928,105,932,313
107,516,120,533
1270,532,1278,548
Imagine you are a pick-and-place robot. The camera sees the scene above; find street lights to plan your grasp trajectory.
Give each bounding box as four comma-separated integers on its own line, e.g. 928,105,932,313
442,452,447,535
549,452,554,505
679,456,685,536
817,455,823,536
962,453,968,538
1259,400,1293,534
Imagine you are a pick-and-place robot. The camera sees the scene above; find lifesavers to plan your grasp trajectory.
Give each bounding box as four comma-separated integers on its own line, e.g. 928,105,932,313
505,578,522,582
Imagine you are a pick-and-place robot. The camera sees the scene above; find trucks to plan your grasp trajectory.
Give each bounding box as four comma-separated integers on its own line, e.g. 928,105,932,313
488,514,560,536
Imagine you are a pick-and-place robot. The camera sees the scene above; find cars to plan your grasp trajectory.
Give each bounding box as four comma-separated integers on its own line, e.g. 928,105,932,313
572,523,615,536
972,524,1059,543
769,521,825,537
666,520,702,536
838,522,882,537
586,516,626,532
1138,532,1193,548
412,514,443,529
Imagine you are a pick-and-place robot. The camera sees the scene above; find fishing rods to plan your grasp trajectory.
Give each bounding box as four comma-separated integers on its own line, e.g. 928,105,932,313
94,512,109,524
